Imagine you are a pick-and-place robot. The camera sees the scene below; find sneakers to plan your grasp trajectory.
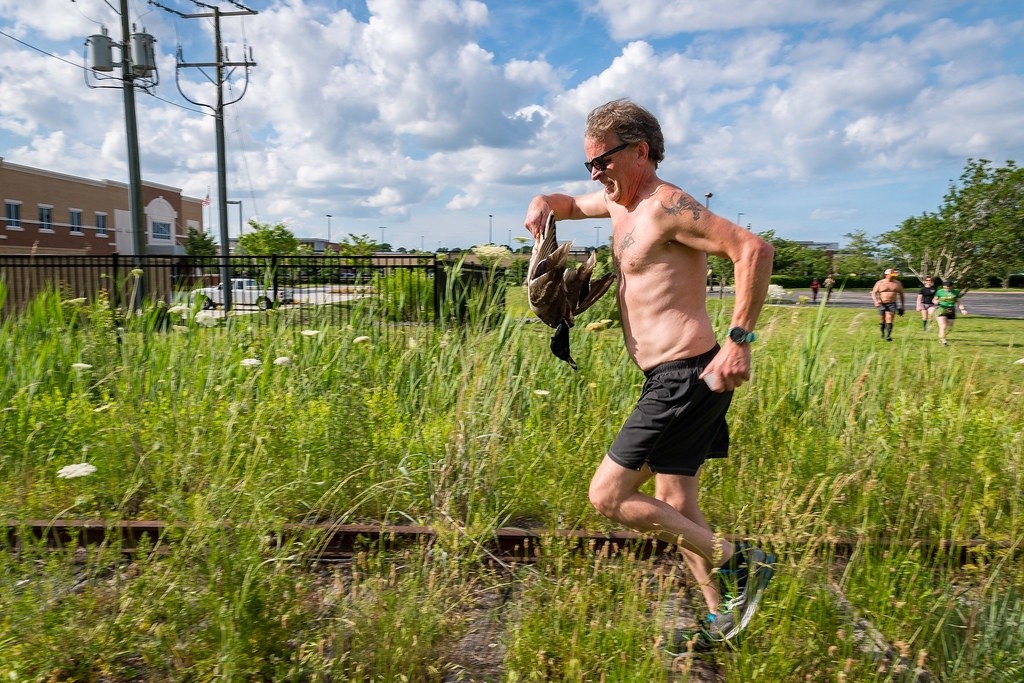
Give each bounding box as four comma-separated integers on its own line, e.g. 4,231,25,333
665,631,727,657
710,542,776,641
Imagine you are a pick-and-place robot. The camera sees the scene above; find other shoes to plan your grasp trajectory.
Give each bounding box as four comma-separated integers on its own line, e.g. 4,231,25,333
887,336,891,341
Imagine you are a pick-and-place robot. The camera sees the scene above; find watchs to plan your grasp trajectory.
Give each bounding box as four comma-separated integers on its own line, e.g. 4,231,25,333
728,327,757,346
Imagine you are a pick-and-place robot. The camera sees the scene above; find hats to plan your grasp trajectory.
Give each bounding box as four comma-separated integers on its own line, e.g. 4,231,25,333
884,269,897,276
943,279,952,286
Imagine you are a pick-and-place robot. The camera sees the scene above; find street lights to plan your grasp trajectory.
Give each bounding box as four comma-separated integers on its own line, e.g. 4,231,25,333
705,192,714,208
737,212,745,225
326,214,333,243
489,214,493,244
594,226,604,248
227,201,243,254
380,226,387,243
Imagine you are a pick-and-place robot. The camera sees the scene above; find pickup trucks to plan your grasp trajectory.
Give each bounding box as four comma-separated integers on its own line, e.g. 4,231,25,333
189,277,294,311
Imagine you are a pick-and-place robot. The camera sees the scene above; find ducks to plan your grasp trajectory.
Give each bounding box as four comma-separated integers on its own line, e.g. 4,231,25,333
525,209,617,371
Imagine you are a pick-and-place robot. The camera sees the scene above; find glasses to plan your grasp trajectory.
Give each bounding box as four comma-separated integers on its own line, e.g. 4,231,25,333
926,280,931,281
584,143,628,171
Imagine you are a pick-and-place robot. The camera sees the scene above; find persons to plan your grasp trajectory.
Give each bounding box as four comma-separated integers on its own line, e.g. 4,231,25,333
872,269,905,341
524,99,775,658
917,277,938,331
932,282,968,346
824,275,835,301
811,278,820,302
